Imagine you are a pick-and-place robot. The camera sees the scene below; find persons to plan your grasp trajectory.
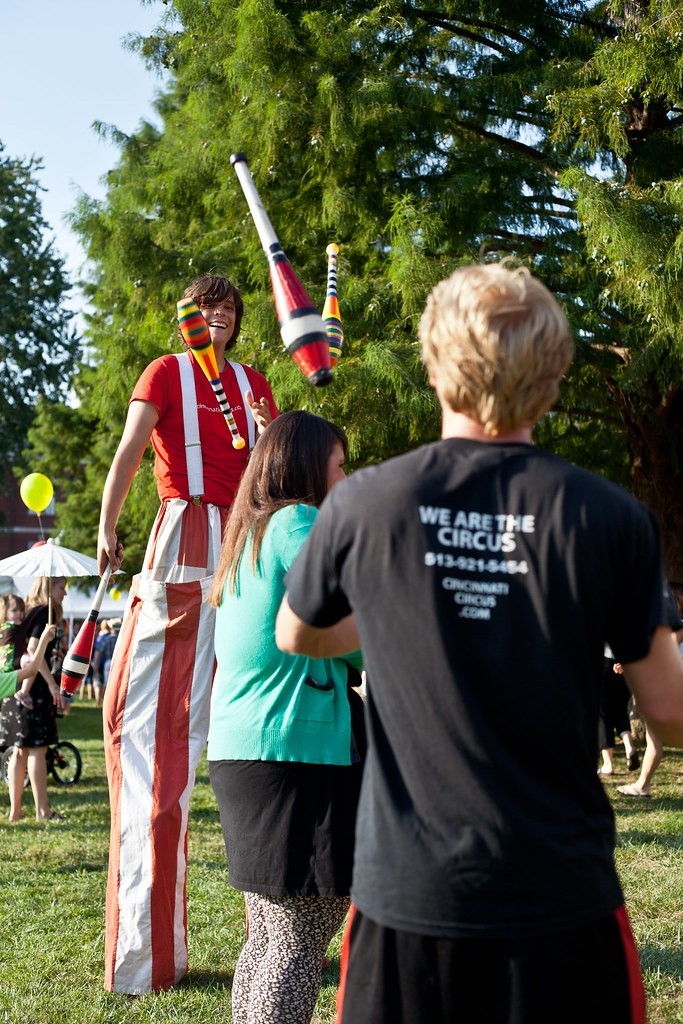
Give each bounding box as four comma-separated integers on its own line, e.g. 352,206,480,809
71,618,123,706
268,261,683,1023
597,640,640,775
0,593,33,708
0,621,57,703
8,576,67,825
96,275,291,770
207,410,369,1024
612,593,683,800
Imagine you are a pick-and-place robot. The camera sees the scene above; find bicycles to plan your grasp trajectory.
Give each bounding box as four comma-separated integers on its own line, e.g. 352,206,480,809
1,708,81,787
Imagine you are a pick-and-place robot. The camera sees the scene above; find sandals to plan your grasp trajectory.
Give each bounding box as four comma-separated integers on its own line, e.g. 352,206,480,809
616,784,652,797
14,689,33,710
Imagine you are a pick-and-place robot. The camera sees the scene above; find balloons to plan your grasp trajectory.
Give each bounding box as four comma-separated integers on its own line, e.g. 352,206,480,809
20,472,54,542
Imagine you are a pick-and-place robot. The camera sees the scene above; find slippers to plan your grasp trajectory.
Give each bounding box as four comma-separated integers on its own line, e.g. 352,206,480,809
597,768,614,776
626,749,640,771
35,811,67,821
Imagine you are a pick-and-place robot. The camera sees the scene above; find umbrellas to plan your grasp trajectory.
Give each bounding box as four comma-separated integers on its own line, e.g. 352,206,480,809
0,537,128,624
